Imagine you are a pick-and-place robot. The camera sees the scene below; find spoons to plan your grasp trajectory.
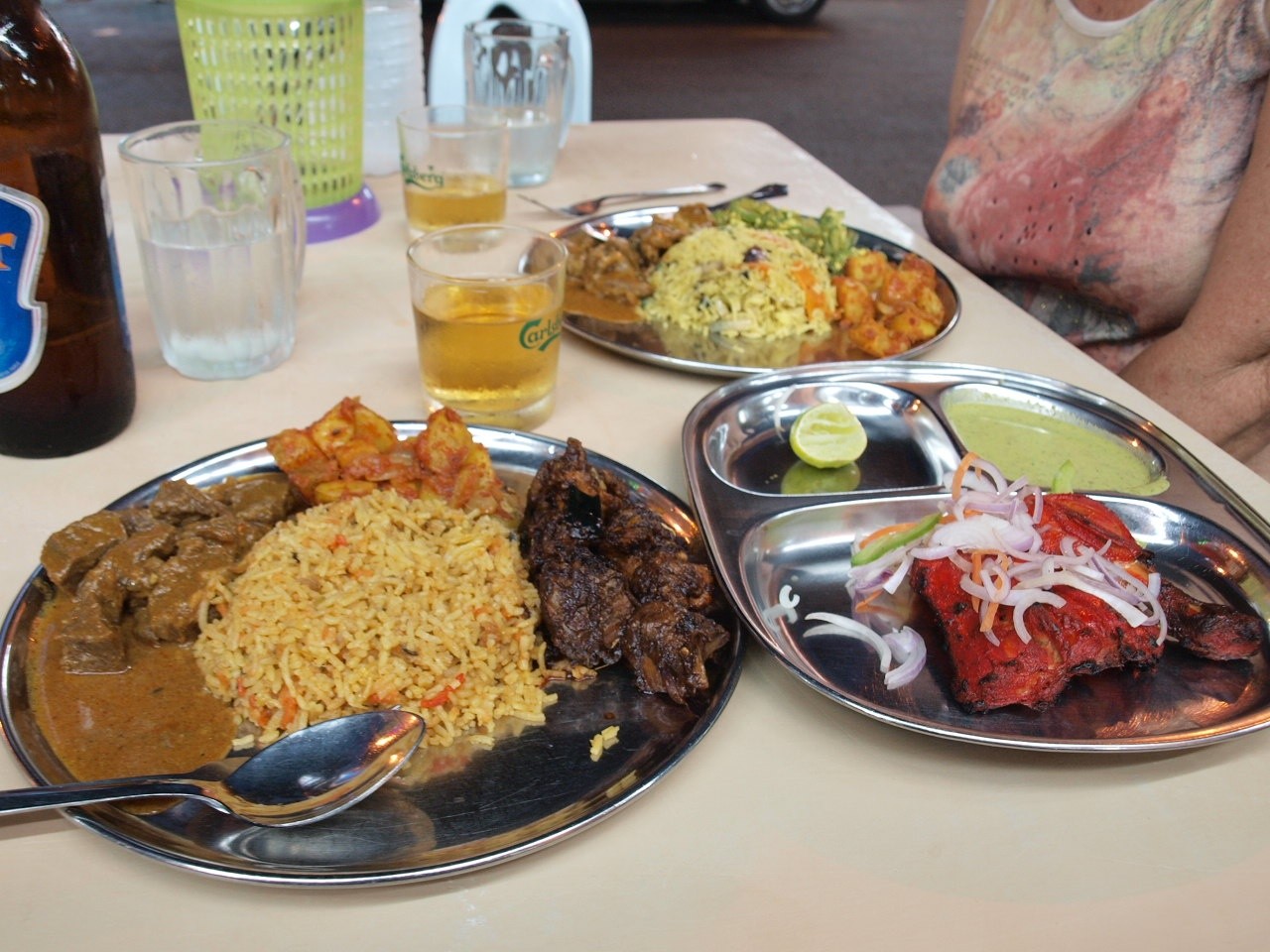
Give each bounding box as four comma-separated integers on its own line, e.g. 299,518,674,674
0,710,425,828
581,181,788,241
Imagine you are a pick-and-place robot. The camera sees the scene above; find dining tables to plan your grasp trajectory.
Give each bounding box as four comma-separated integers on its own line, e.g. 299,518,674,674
0,120,1267,949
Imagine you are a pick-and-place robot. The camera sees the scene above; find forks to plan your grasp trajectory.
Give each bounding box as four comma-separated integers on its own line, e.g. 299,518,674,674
519,181,727,221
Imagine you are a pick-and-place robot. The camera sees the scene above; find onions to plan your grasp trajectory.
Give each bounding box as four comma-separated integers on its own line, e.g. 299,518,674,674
785,447,1169,696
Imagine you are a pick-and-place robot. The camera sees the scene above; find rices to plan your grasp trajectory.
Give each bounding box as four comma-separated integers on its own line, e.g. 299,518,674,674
640,216,835,352
193,487,620,786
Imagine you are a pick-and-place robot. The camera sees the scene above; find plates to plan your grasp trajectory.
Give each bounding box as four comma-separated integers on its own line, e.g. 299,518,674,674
1,421,755,887
517,206,963,377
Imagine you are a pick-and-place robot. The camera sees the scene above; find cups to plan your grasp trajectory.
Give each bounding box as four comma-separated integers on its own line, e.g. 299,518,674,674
117,118,306,381
396,102,515,269
404,223,566,436
463,19,576,189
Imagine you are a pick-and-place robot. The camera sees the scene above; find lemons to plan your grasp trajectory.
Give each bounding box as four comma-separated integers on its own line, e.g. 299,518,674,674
789,399,869,471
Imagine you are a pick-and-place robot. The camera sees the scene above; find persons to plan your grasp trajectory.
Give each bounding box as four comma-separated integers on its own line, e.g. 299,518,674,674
921,0,1269,480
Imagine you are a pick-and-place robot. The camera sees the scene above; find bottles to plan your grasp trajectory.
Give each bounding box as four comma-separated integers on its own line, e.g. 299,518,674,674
2,0,138,463
